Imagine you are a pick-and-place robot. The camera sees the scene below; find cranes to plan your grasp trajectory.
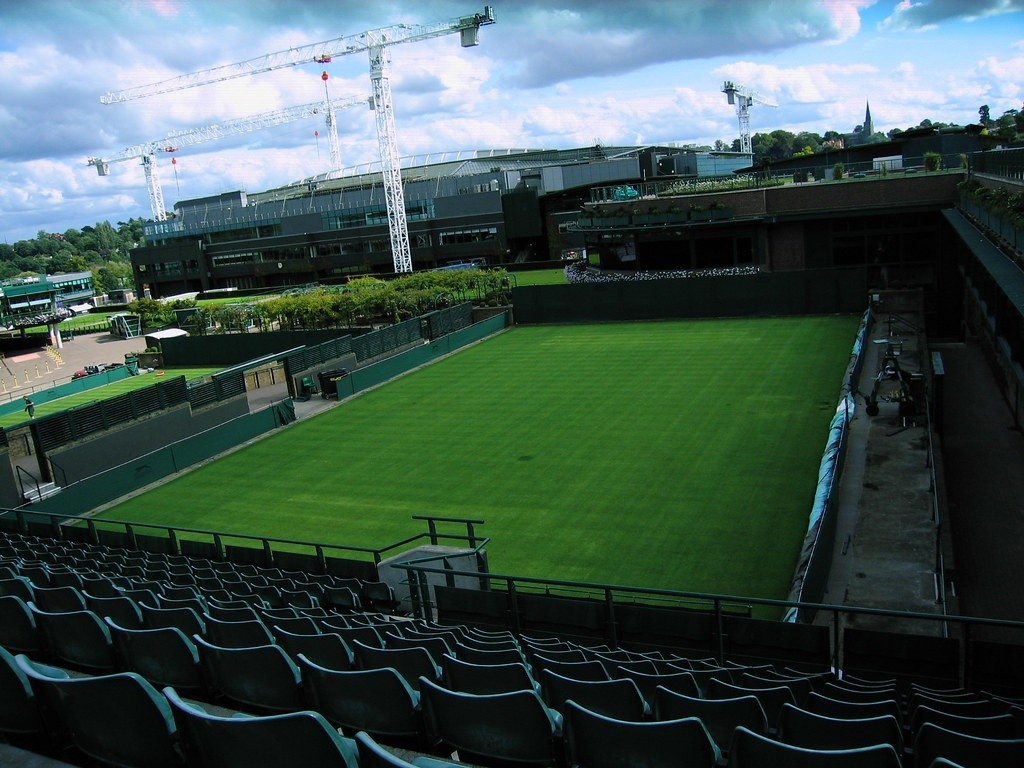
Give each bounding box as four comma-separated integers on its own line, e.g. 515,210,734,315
84,92,370,232
98,4,498,273
720,78,779,156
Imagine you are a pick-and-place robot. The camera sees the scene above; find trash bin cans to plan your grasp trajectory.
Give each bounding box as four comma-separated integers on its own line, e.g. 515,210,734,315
317,367,349,400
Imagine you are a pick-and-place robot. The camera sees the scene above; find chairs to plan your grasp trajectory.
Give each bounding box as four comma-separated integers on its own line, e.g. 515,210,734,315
303,377,319,395
0,532,1024,768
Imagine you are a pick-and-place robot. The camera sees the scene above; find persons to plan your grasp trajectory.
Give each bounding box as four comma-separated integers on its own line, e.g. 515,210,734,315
23,396,35,419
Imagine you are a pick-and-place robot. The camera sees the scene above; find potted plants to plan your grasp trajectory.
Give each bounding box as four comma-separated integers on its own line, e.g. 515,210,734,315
923,150,942,172
834,163,845,179
